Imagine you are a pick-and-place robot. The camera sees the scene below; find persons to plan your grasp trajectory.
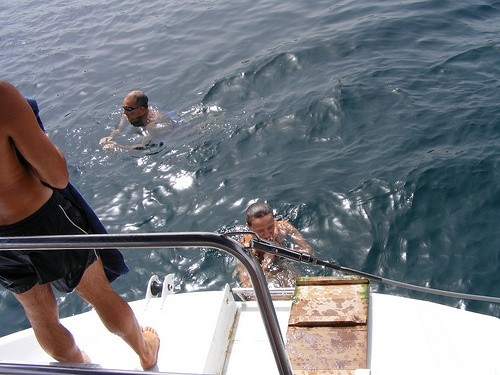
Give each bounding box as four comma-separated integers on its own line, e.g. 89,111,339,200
100,89,174,154
1,80,160,370
235,201,313,301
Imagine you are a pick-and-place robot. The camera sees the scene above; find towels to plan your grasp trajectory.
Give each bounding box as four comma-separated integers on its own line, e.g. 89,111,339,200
21,92,130,285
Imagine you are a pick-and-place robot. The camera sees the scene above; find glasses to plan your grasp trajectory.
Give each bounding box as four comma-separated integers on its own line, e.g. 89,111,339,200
122,105,140,112
